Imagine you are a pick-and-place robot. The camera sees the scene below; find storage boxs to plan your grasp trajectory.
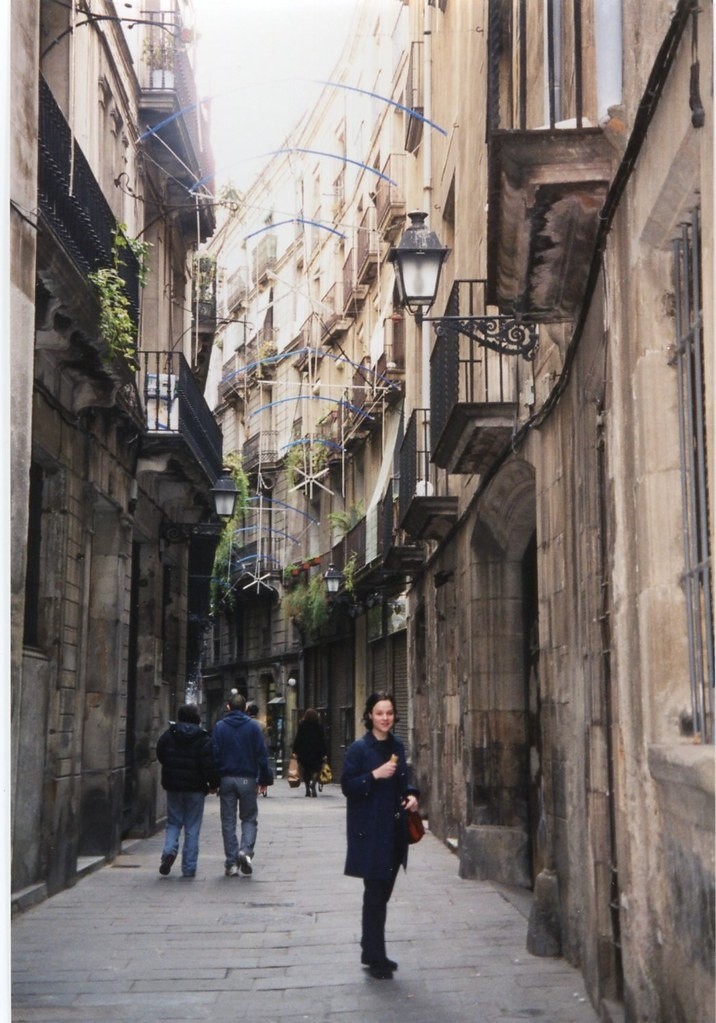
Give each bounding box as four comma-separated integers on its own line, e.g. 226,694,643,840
147,372,179,432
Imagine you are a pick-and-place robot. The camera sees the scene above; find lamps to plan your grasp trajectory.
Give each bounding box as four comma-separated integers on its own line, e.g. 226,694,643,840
434,569,455,591
280,676,297,688
158,463,243,564
231,682,238,695
385,206,540,363
322,561,382,598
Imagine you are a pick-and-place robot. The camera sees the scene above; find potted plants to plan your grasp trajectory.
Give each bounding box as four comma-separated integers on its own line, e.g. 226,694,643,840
282,443,326,490
283,550,320,579
144,24,187,93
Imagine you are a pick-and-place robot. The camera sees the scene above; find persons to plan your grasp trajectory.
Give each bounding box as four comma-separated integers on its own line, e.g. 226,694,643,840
156,704,213,876
341,692,420,980
247,704,267,797
292,709,328,797
211,694,273,877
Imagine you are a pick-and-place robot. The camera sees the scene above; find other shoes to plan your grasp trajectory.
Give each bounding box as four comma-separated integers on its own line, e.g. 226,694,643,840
182,871,193,877
225,864,238,877
237,849,252,874
159,854,175,875
367,956,397,979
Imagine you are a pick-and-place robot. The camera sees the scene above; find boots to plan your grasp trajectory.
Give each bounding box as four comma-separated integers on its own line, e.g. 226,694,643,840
310,784,316,797
305,783,310,797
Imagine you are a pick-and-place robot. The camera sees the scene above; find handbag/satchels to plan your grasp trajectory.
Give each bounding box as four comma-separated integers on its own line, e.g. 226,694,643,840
318,764,331,792
286,756,300,788
403,797,424,844
